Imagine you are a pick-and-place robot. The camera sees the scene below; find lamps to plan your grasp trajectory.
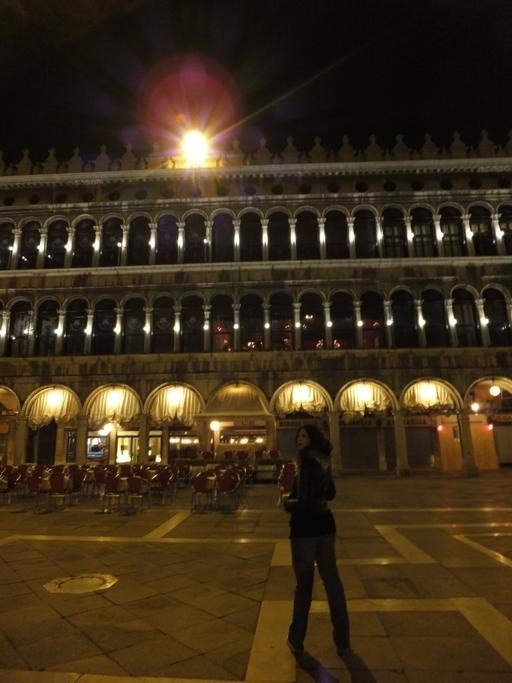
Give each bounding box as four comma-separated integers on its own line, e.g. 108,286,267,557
488,379,501,397
470,391,480,411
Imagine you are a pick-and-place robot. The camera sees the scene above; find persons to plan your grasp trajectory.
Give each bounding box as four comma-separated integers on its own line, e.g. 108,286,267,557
282,423,349,656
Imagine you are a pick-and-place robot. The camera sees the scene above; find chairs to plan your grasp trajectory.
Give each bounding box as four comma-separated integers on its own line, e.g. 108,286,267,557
1,455,298,510
